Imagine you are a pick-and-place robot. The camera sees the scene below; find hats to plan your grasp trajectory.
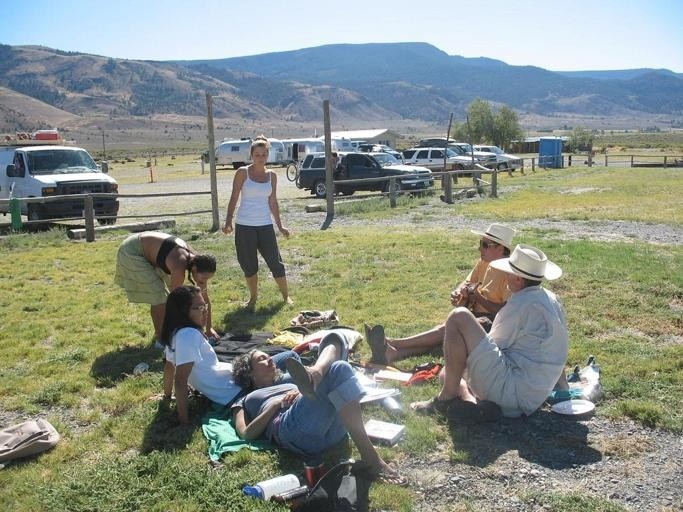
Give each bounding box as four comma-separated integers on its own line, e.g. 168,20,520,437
487,244,563,282
470,223,516,249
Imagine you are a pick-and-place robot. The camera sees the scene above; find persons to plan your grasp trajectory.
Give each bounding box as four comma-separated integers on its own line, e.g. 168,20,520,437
156,286,300,425
112,230,213,351
228,330,407,486
409,242,570,419
363,222,519,363
221,135,297,306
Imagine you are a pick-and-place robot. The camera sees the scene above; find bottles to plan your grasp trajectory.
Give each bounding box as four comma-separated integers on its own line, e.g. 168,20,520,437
383,394,407,421
243,472,309,505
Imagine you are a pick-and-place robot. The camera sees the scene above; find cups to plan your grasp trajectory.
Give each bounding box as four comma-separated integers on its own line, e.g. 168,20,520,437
304,456,327,489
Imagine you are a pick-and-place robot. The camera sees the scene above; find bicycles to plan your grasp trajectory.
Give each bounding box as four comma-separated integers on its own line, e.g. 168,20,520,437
285,158,303,190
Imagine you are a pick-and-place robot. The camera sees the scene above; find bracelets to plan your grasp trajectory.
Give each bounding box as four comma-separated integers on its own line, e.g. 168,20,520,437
163,393,170,399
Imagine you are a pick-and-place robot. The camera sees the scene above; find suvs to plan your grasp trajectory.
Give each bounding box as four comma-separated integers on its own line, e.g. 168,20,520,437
200,150,209,163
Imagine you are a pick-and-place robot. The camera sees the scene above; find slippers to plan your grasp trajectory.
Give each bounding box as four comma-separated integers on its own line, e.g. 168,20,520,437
365,323,372,350
410,397,460,414
371,325,389,366
362,462,409,487
285,355,319,403
445,400,482,425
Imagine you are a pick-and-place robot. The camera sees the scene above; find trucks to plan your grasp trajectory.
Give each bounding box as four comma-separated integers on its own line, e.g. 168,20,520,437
297,136,526,197
0,144,119,232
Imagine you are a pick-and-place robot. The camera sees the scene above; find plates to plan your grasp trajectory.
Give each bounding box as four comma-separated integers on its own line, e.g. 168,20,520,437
552,399,595,415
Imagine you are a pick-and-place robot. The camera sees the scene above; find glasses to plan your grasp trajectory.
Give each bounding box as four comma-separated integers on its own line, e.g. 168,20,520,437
192,303,209,311
478,238,498,248
